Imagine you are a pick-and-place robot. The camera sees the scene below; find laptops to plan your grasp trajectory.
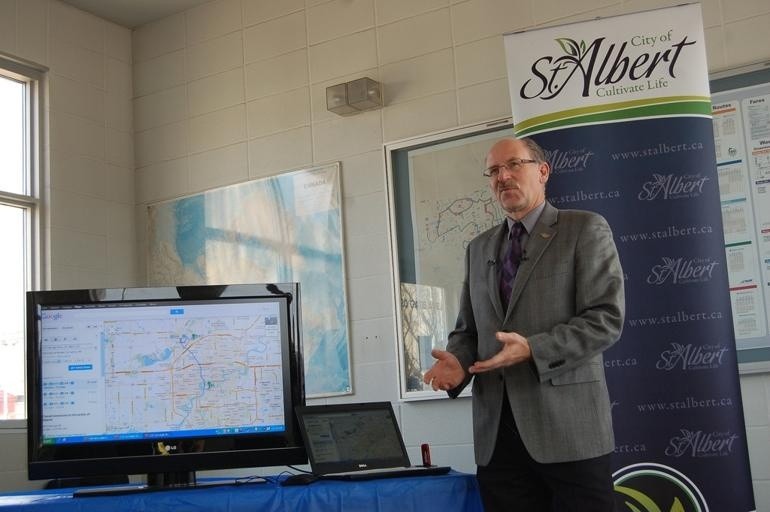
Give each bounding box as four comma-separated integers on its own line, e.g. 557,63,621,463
293,401,453,481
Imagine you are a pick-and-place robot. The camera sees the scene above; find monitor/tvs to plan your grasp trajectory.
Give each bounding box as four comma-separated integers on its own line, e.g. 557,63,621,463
24,280,303,496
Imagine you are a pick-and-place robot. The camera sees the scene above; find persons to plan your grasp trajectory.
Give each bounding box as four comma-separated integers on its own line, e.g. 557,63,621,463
423,134,627,512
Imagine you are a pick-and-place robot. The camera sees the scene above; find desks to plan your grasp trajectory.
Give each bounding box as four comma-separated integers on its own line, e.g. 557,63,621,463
3,450,485,512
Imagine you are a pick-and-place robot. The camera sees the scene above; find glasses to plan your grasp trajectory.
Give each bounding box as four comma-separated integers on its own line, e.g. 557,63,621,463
481,157,536,178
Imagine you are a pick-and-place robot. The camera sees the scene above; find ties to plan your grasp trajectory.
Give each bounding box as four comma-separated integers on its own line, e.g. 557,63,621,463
498,221,524,315
